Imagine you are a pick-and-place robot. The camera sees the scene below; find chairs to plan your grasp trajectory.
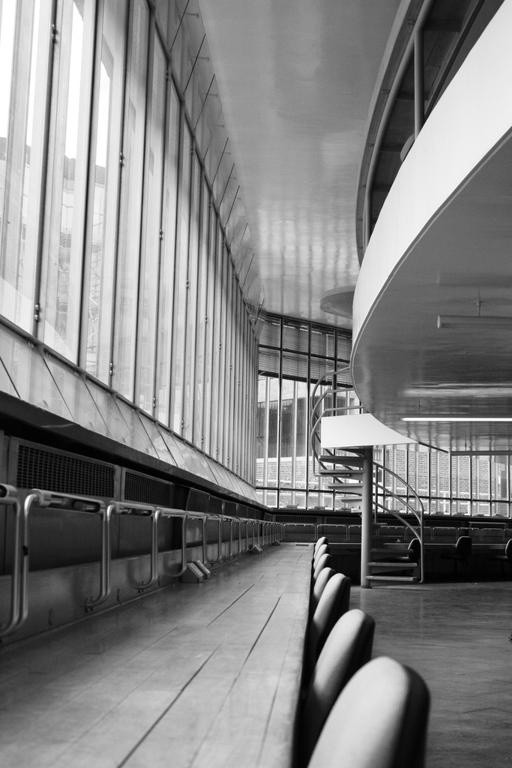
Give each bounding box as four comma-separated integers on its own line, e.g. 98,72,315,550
431,524,510,576
301,535,431,767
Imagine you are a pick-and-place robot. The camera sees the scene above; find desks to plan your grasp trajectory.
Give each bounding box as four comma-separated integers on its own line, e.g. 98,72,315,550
3,533,311,768
